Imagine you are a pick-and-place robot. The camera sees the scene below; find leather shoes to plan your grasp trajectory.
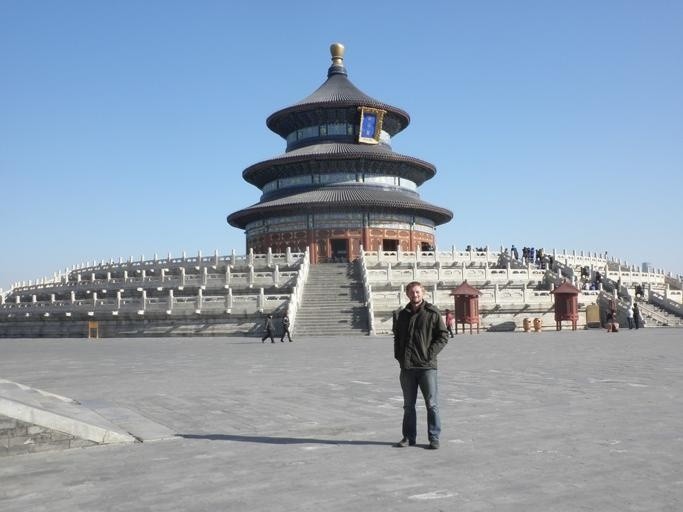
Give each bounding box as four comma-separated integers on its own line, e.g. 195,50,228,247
398,437,415,447
429,440,440,449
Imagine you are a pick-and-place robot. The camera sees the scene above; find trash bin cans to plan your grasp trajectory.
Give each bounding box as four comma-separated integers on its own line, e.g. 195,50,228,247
523,318,531,333
534,318,542,333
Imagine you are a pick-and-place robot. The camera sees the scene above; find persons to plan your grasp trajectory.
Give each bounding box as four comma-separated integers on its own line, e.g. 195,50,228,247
466,245,490,256
504,245,554,269
262,315,277,343
279,312,293,343
445,309,454,338
422,242,435,256
627,301,640,330
581,267,621,299
393,282,455,449
